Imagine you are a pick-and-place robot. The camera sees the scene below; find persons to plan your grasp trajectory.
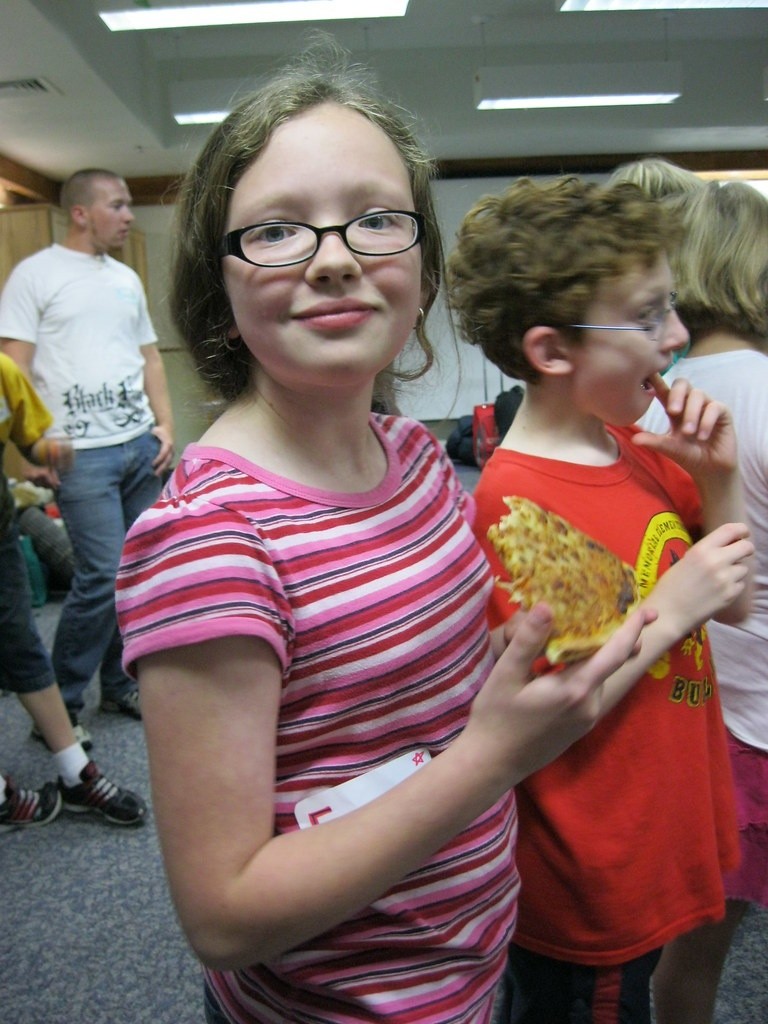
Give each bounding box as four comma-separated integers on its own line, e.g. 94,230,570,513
0,351,147,826
114,56,645,1023
448,154,768,1023
1,170,175,752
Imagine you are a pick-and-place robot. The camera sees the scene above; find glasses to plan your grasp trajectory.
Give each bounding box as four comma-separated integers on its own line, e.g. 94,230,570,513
217,210,426,267
549,290,679,341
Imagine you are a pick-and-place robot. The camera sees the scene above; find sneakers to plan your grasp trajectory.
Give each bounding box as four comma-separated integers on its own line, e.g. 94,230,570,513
97,688,141,721
30,712,92,753
0,775,63,833
57,760,147,824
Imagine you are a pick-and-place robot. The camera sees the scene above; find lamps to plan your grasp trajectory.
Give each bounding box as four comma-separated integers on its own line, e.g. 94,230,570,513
468,18,685,112
170,23,372,127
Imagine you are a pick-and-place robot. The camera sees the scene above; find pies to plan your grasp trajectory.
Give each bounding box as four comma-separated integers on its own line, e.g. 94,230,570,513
485,495,641,665
